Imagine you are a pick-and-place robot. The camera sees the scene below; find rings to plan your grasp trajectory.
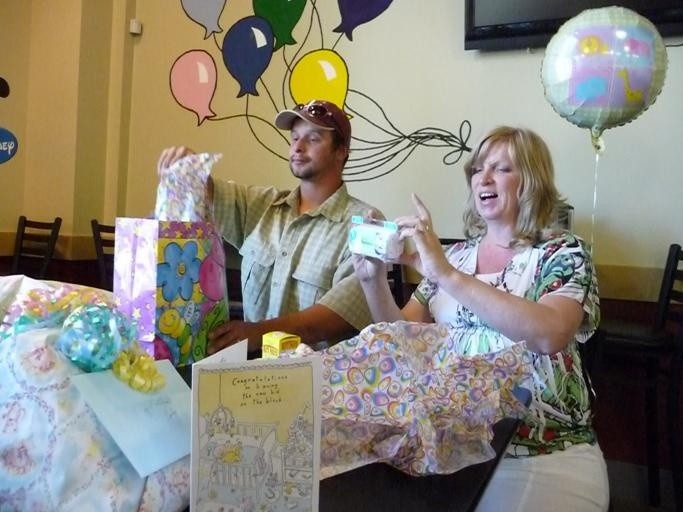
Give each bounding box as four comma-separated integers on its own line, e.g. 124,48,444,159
425,224,429,231
236,339,241,343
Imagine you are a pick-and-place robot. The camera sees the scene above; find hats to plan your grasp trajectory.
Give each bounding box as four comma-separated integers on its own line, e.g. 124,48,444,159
275,98,351,149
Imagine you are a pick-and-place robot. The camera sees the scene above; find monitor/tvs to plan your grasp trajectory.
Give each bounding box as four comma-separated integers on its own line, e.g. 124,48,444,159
464,0,682,52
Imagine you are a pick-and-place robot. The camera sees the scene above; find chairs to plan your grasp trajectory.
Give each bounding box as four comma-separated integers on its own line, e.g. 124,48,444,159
10,213,115,283
575,244,682,510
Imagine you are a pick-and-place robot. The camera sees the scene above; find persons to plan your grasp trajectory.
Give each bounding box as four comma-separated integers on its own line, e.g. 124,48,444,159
151,98,389,359
346,126,613,511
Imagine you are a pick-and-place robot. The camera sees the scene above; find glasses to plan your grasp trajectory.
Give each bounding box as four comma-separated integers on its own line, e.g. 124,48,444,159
291,103,346,141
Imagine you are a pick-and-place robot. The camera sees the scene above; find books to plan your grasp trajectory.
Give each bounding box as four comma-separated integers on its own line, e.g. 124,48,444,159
187,338,322,512
110,216,229,370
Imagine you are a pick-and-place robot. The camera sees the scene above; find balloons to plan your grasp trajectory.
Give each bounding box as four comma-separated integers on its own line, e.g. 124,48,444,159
168,50,219,126
219,13,275,100
180,0,226,41
330,0,394,43
538,5,670,153
287,48,353,121
250,2,307,54
